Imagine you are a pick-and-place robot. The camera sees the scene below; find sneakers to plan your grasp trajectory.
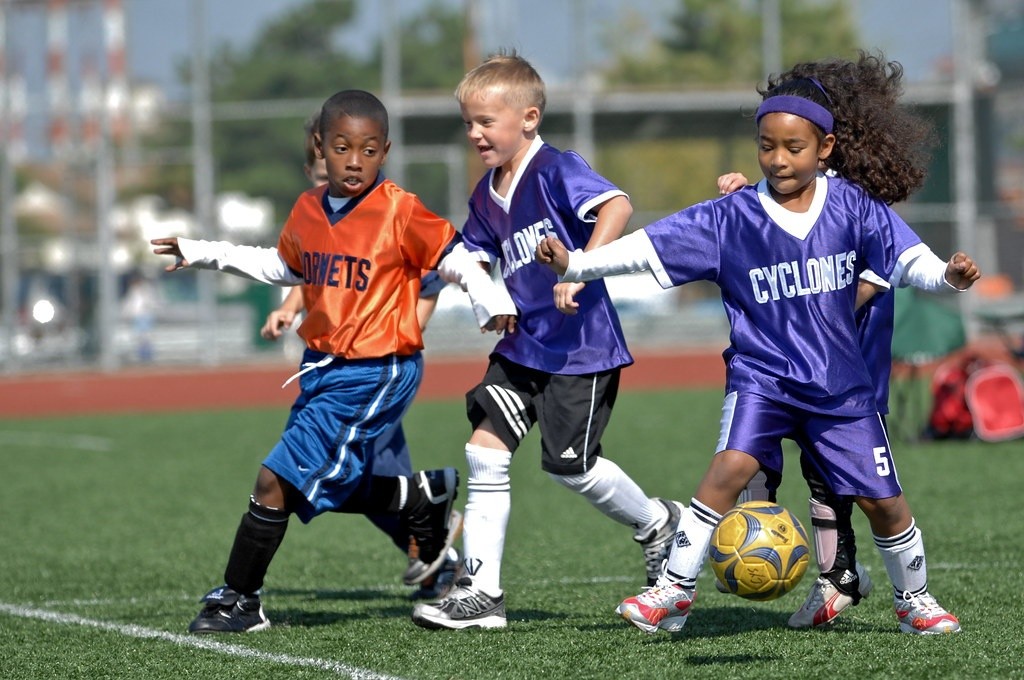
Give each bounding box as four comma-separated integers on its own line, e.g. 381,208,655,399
407,545,464,601
632,497,684,589
895,589,963,635
408,468,460,564
615,559,697,635
188,584,271,633
402,509,463,585
787,563,874,628
412,576,508,629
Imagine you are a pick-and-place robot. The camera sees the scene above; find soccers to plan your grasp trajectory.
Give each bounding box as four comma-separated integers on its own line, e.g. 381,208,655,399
709,501,810,601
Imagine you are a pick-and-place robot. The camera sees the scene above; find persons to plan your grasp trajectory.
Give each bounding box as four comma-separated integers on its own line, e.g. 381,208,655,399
531,50,984,639
715,116,937,632
259,110,460,597
412,48,694,629
150,88,518,637
